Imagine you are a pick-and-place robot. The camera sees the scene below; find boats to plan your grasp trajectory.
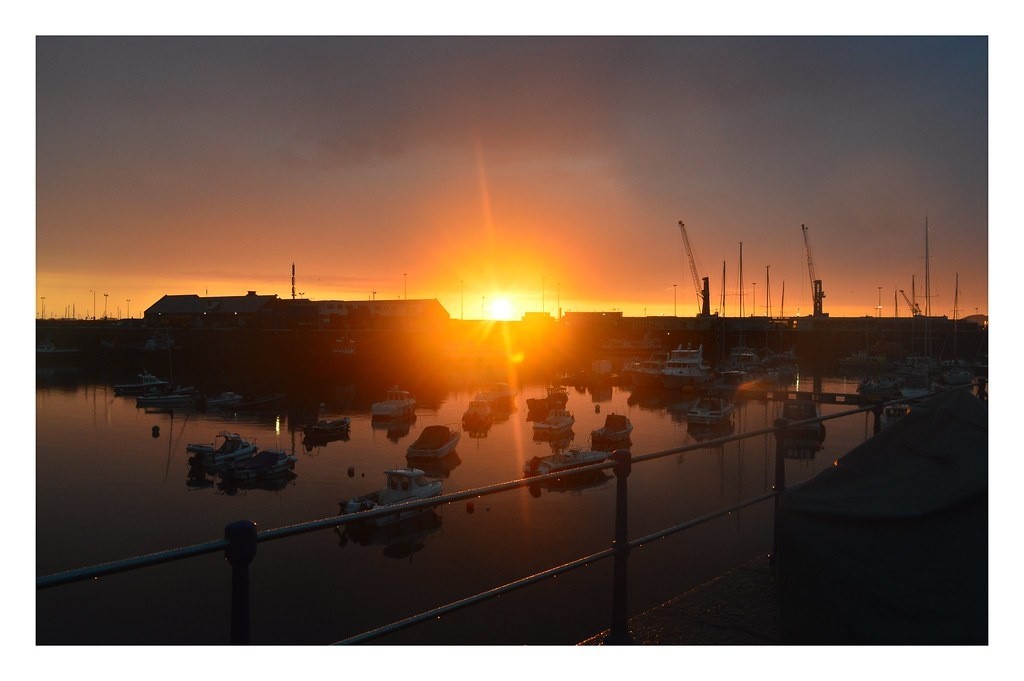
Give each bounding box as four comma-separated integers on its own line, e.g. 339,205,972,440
532,408,575,434
526,387,568,408
339,464,442,525
185,429,260,472
624,359,660,381
136,386,189,404
303,417,352,437
463,400,492,420
477,382,515,409
773,399,826,439
371,385,417,422
839,349,882,376
524,445,611,477
406,423,462,461
219,446,298,482
37,337,83,354
592,414,632,442
661,344,716,390
878,404,910,431
208,391,242,407
114,373,168,395
687,396,734,425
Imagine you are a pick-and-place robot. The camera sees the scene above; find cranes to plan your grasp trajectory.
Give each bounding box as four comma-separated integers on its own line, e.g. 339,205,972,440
801,224,826,301
678,220,710,299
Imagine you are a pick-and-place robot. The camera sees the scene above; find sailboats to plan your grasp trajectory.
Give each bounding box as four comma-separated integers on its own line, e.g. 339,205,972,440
163,331,196,395
896,277,988,385
718,241,796,385
